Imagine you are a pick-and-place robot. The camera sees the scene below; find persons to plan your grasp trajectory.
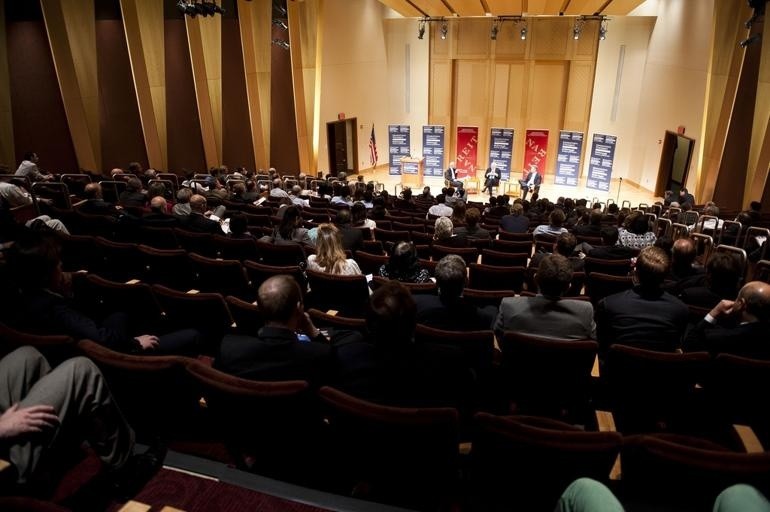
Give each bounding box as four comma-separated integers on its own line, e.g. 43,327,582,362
555,478,769,512
0,345,135,511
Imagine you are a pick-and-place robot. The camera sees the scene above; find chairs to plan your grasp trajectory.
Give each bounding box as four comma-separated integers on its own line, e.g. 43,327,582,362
0,161,769,512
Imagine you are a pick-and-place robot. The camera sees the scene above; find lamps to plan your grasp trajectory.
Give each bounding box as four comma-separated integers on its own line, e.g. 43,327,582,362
418,16,448,40
490,16,528,40
572,14,613,41
272,2,291,52
741,3,766,47
174,0,227,20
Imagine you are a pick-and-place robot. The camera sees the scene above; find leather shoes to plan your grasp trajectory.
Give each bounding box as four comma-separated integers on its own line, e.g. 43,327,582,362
112,448,163,504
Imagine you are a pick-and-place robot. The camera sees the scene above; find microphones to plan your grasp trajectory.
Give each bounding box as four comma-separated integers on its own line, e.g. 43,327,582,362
620,177,622,180
